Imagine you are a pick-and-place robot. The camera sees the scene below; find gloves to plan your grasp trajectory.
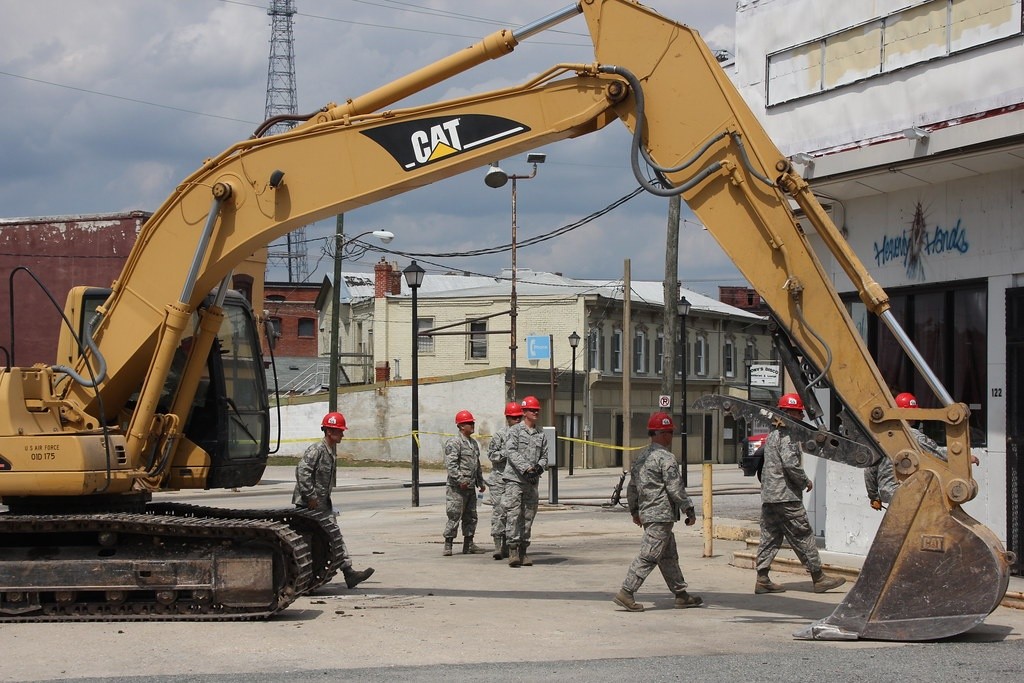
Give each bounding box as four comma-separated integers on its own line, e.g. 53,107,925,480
527,472,539,485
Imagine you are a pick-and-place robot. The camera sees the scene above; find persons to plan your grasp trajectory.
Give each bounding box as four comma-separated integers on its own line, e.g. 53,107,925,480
291,412,375,589
488,396,548,566
613,412,703,612
443,411,486,555
755,392,844,594
864,392,979,512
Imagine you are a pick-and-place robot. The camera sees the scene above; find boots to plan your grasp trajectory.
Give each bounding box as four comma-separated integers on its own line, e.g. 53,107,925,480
754,574,785,593
813,570,846,593
492,535,505,560
443,537,453,556
519,547,532,566
463,535,485,554
508,546,520,566
613,588,643,612
345,567,374,589
675,592,703,609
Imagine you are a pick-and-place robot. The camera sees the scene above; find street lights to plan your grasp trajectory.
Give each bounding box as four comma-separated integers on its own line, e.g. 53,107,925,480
402,260,426,507
743,355,754,400
677,296,691,485
567,331,581,475
328,229,394,488
485,153,546,402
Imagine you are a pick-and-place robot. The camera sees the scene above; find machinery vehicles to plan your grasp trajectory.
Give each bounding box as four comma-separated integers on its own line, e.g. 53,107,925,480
0,0,1016,640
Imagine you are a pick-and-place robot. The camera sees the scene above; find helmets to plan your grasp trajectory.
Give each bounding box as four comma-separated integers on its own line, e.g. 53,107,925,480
321,412,348,430
895,393,919,408
521,396,541,409
504,402,524,416
456,410,476,424
777,393,805,409
647,412,677,430
